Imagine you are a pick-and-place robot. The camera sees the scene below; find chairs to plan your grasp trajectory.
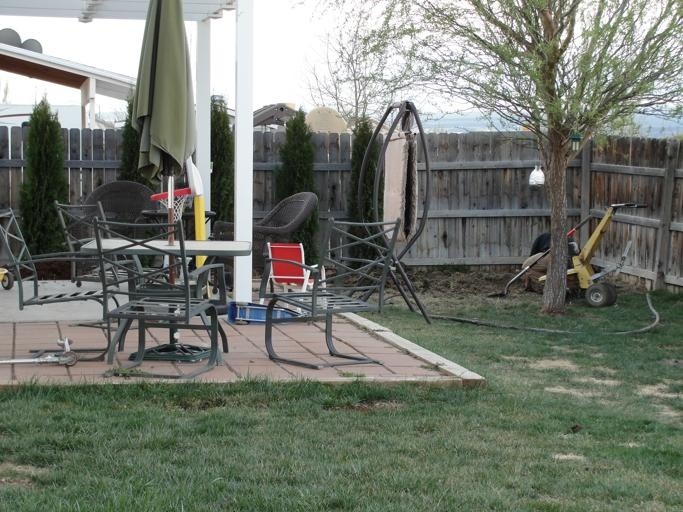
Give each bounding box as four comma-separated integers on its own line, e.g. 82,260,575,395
1,181,401,377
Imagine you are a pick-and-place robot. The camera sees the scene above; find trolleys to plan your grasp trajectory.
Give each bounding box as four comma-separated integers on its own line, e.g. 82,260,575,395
534,203,644,307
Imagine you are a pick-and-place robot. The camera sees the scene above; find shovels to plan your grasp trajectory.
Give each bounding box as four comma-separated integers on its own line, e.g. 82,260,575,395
488,214,594,298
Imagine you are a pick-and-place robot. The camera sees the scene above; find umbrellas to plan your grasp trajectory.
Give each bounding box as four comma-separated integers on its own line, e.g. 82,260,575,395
130,0,197,345
